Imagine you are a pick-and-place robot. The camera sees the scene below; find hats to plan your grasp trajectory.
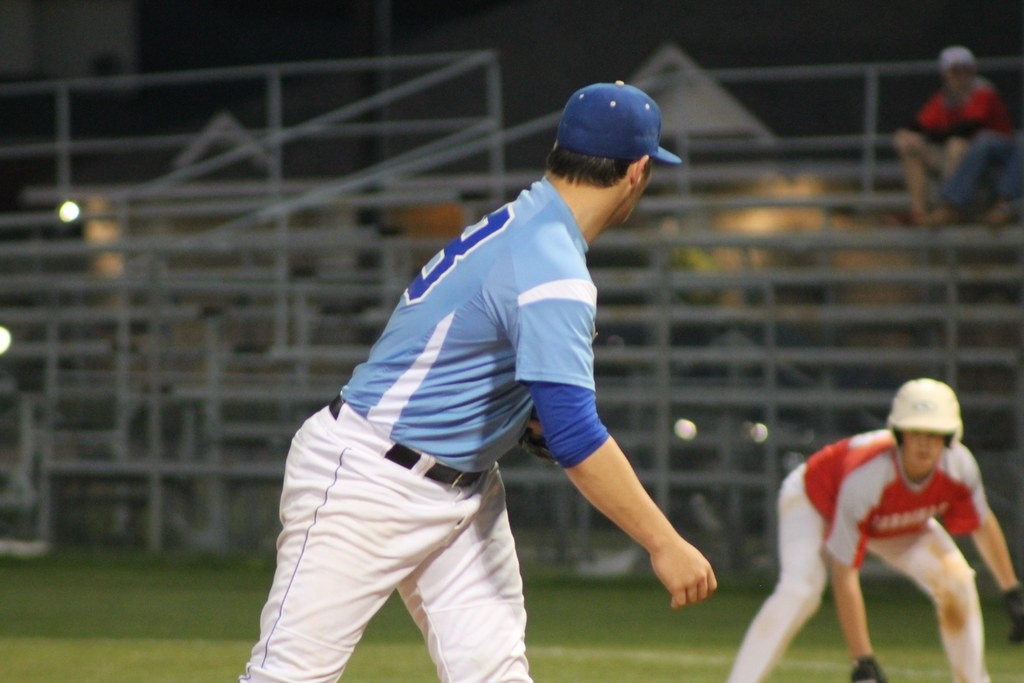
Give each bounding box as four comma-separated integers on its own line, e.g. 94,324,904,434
558,79,682,163
936,46,977,76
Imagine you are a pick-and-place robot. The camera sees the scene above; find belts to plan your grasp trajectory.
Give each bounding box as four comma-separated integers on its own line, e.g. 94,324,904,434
329,394,480,488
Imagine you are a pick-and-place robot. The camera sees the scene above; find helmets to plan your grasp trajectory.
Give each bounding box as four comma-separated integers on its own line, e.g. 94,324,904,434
887,378,963,451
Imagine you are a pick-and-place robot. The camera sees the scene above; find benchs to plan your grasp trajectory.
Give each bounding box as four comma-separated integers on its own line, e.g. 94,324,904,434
0,52,1024,575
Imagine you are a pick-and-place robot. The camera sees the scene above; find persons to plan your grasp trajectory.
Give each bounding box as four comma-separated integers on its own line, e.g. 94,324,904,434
727,379,1024,683
241,85,718,683
893,46,1011,225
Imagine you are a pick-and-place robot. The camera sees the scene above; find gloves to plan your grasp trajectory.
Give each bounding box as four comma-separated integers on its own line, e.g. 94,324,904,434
1000,584,1024,643
851,657,884,682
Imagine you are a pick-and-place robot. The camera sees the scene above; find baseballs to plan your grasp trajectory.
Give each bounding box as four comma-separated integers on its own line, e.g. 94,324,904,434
673,417,697,443
748,422,770,444
